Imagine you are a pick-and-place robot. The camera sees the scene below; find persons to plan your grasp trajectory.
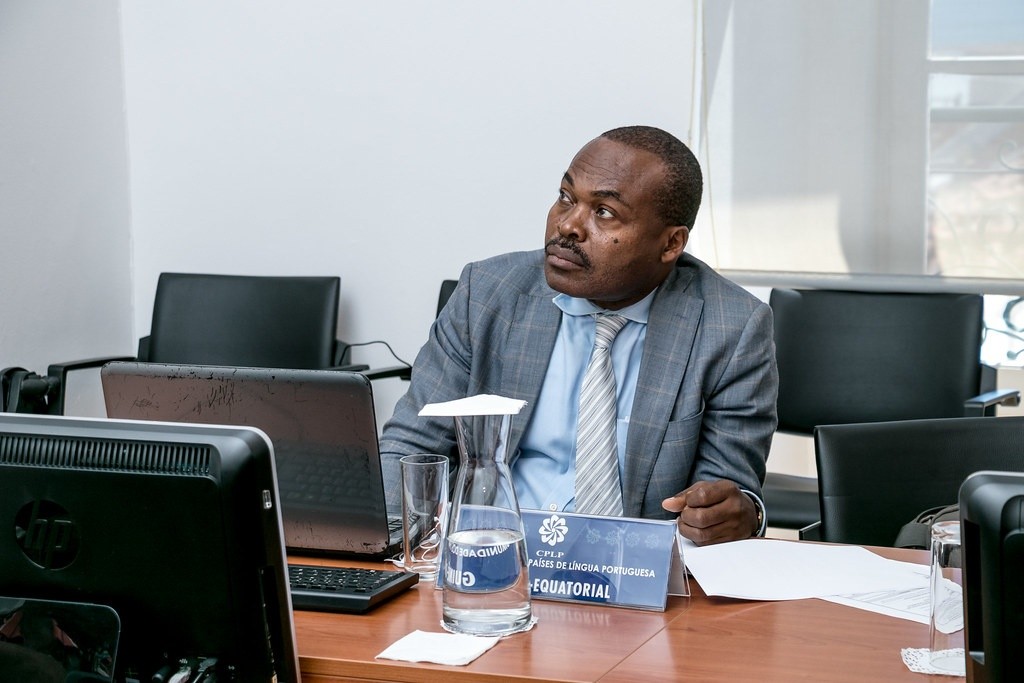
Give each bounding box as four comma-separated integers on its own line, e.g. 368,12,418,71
379,124,779,547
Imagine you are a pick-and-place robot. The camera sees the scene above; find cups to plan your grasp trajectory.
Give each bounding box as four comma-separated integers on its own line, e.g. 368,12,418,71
400,454,450,581
927,518,965,675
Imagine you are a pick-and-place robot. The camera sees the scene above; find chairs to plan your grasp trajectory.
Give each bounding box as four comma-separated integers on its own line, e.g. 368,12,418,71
47,272,351,417
799,415,1024,543
759,284,1024,530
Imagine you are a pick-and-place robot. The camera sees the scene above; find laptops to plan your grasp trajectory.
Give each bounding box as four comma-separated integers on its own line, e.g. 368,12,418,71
102,360,425,563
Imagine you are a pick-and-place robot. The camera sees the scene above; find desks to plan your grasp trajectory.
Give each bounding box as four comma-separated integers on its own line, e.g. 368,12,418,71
283,534,969,683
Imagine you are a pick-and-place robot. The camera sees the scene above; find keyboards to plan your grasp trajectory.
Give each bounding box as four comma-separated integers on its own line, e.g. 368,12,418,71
286,564,420,615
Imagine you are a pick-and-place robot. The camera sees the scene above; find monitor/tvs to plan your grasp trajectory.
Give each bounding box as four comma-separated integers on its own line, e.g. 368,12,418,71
958,470,1024,683
0,413,299,683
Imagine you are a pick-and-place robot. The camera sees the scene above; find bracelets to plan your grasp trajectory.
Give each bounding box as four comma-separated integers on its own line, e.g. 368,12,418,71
741,490,767,538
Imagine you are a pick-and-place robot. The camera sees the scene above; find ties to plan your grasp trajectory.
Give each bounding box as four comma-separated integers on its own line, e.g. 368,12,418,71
575,313,630,518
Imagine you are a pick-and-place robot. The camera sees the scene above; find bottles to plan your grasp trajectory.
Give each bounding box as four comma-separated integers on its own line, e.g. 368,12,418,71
443,414,533,637
